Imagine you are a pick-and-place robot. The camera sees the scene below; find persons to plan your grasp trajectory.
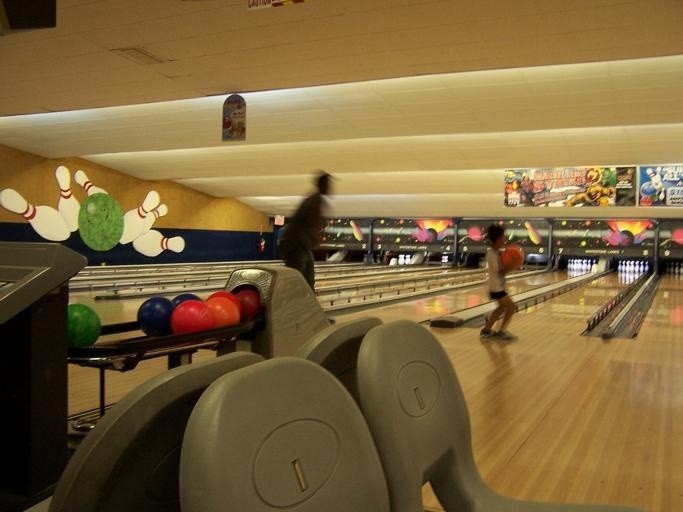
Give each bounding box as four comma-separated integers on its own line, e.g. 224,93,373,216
279,171,334,293
480,224,518,338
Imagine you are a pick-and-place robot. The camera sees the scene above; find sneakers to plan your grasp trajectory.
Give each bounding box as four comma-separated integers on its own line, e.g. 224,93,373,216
480,330,516,341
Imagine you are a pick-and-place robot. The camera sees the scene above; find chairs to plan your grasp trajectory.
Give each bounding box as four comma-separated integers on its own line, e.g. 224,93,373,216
356,320,652,512
298,315,387,415
177,354,394,512
46,351,267,512
